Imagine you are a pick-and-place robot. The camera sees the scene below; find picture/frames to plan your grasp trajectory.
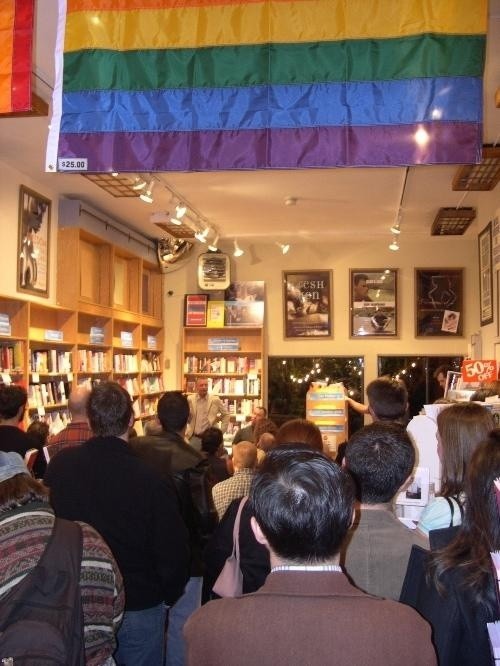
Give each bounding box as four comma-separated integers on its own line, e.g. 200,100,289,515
477,219,494,327
413,265,466,339
281,267,333,340
15,181,53,299
183,291,210,326
348,265,402,339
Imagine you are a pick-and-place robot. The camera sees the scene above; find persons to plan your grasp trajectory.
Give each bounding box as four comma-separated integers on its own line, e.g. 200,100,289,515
370,312,394,333
1,363,500,666
352,273,373,301
445,312,457,331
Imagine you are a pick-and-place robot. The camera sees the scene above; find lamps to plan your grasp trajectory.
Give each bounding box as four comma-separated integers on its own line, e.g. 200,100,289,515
275,240,290,254
388,213,405,252
231,239,243,256
110,168,226,253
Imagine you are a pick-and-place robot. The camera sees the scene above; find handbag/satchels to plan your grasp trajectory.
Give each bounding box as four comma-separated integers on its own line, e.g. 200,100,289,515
1,518,85,665
211,556,246,599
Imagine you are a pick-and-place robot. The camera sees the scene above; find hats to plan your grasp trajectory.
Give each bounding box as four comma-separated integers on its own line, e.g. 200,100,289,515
0,450,33,484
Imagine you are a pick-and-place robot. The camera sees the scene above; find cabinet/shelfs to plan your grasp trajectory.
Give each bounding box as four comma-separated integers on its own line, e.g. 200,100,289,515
1,290,165,436
304,384,350,460
180,324,266,447
55,225,165,328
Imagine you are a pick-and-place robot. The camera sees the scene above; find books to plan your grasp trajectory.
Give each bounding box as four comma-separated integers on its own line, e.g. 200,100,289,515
183,353,267,444
1,312,166,443
